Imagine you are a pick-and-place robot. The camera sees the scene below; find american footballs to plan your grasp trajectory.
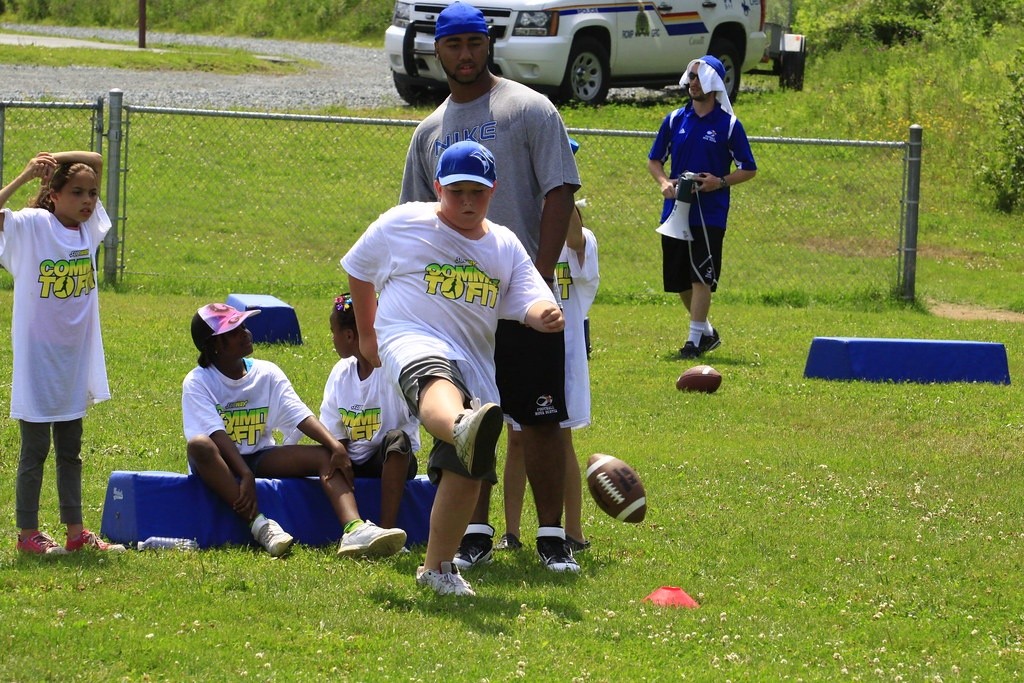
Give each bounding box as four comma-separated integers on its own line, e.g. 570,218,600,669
583,453,650,526
675,363,723,396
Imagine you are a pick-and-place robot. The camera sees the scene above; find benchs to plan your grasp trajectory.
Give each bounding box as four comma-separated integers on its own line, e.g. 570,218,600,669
226,294,304,346
802,337,1011,386
99,470,436,550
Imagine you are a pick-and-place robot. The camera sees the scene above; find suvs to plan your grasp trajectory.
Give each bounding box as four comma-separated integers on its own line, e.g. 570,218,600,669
381,0,769,109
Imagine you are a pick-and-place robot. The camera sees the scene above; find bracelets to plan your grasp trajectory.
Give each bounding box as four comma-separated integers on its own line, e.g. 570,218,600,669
720,177,728,188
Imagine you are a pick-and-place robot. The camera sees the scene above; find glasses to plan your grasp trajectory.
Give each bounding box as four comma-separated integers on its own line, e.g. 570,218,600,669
688,71,701,80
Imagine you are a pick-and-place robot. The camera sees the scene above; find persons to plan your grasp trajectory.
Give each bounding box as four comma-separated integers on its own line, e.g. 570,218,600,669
341,138,565,595
319,292,420,553
492,206,600,554
647,56,757,359
399,0,582,571
182,303,407,556
0,151,125,556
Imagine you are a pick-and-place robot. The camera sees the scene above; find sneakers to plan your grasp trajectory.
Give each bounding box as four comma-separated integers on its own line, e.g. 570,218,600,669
536,525,580,571
680,332,708,357
452,523,495,570
415,561,476,596
17,529,69,554
251,515,294,557
703,328,721,352
65,529,126,553
453,391,504,477
337,520,408,558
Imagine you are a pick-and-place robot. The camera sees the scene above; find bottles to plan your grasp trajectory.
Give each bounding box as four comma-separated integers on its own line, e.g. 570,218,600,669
137,537,195,553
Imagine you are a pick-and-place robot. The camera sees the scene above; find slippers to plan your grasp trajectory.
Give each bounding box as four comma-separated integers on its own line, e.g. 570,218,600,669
565,534,590,552
495,533,521,550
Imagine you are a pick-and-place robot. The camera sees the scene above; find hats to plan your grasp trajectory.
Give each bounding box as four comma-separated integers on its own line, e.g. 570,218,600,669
434,0,489,41
436,139,496,188
699,54,726,80
191,302,262,351
